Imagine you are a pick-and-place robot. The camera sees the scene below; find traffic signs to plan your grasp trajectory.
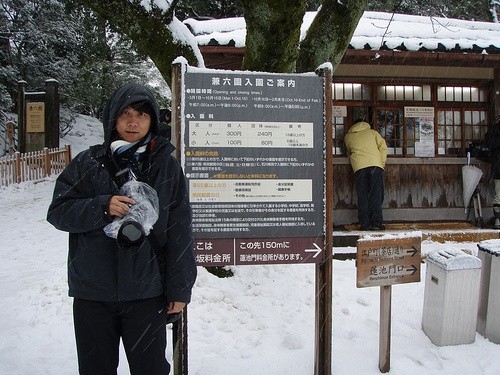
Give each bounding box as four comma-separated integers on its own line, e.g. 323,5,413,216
356,230,422,289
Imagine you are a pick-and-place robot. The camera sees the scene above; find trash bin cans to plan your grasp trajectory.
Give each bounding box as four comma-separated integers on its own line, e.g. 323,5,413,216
422,249,484,347
475,239,500,345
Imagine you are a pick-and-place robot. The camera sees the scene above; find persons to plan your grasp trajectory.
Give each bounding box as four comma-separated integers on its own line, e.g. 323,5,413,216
46,85,197,375
485,119,500,229
344,118,387,230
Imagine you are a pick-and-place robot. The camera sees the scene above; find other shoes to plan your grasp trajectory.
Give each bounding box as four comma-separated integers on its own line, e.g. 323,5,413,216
359,222,386,231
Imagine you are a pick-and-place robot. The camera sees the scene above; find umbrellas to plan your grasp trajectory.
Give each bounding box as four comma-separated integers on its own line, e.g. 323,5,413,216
462,152,483,214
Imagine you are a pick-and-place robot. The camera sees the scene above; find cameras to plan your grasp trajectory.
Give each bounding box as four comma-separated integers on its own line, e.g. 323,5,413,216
116,196,155,249
